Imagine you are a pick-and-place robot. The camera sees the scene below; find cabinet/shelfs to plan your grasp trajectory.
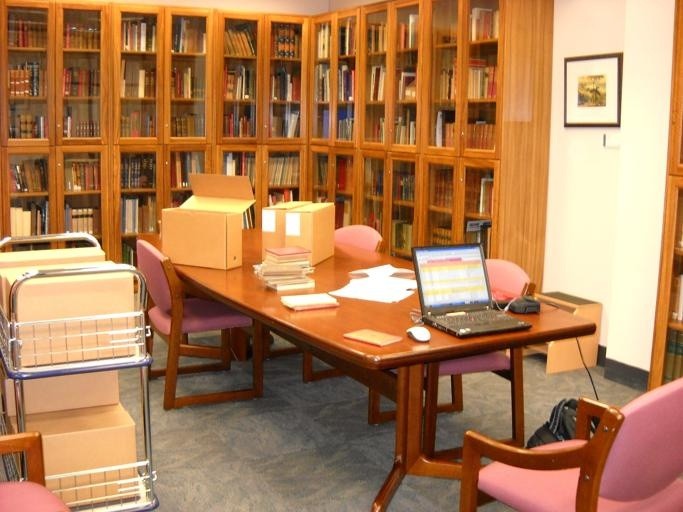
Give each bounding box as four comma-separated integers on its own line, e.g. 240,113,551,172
506,291,603,375
0,2,113,263
644,0,683,391
310,1,554,291
116,5,218,265
563,50,625,129
218,12,308,213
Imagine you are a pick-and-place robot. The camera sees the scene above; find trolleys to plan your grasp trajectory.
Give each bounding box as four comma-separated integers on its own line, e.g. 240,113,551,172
0,232,159,511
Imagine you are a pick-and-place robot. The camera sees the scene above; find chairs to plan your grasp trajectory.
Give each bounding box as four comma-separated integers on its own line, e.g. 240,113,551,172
365,255,539,461
262,224,388,383
458,375,683,512
138,237,264,410
0,430,72,512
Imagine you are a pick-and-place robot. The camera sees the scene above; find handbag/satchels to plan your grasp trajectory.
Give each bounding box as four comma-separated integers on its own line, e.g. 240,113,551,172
526,399,601,450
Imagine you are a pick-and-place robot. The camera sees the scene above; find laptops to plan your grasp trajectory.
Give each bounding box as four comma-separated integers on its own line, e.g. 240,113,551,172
411,243,532,339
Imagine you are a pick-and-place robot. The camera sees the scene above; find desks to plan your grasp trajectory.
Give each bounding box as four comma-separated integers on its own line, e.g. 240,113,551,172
132,225,597,512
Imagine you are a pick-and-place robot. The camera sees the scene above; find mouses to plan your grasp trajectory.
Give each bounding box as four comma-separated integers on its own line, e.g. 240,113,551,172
406,327,431,342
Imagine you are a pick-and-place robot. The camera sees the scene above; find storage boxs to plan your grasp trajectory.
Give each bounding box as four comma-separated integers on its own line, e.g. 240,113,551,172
1,244,141,508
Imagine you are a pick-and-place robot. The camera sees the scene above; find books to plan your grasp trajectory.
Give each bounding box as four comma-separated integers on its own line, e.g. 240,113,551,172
656,273,683,387
427,8,500,259
223,22,300,230
120,14,205,267
316,16,357,228
8,11,102,252
344,327,405,349
250,245,338,311
360,14,418,261
